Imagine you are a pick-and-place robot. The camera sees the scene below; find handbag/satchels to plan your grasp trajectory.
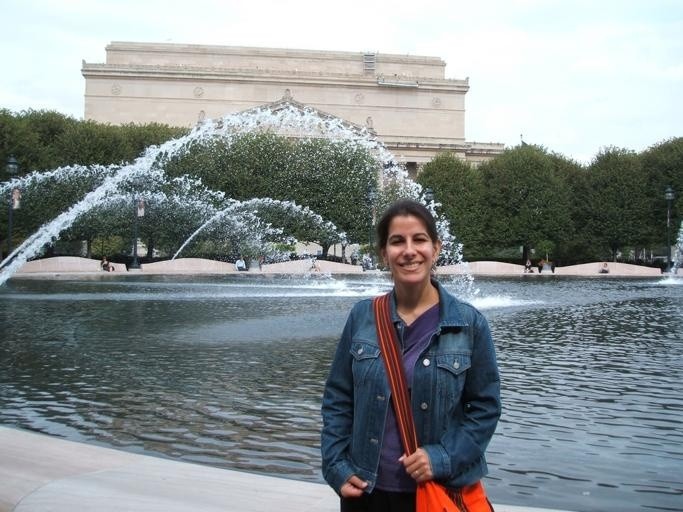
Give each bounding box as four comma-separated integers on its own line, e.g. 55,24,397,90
415,479,495,512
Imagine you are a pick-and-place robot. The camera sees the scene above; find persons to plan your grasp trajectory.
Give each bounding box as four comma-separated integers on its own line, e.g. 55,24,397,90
599,263,609,273
101,256,114,272
236,255,248,271
525,258,532,270
551,262,556,273
538,259,545,273
321,199,502,512
